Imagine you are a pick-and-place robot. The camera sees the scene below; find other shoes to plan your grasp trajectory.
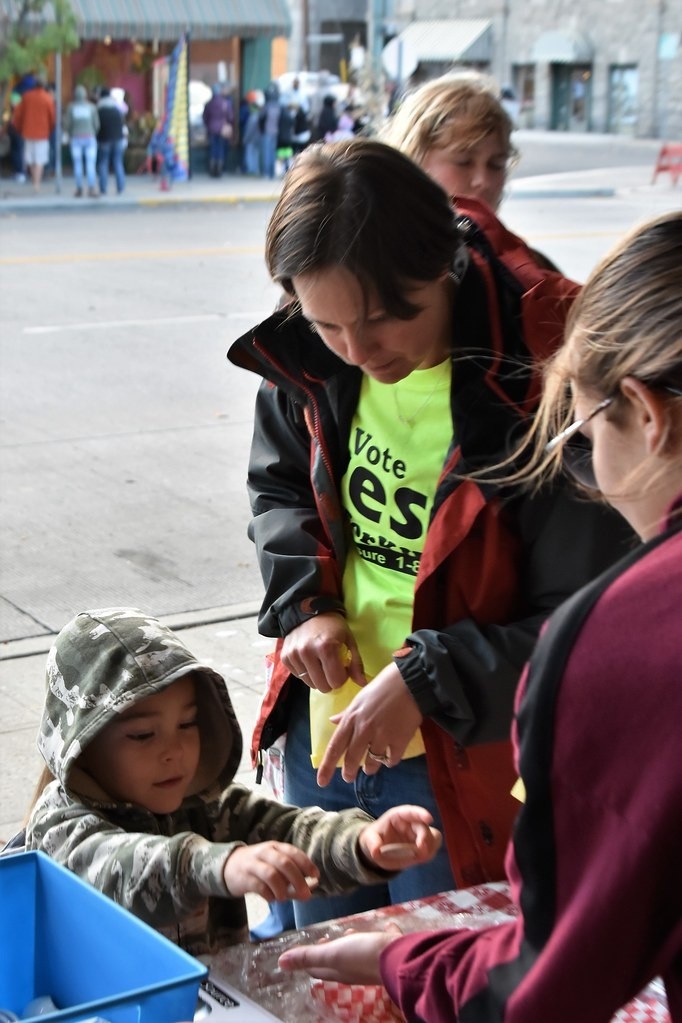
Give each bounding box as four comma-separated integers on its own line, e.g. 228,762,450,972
88,189,101,197
77,189,84,197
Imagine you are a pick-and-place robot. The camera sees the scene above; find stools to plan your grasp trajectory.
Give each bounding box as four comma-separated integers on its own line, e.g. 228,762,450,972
652,141,682,188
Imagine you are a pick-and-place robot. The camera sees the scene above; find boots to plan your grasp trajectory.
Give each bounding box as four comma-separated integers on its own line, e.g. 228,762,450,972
210,157,216,176
217,160,224,178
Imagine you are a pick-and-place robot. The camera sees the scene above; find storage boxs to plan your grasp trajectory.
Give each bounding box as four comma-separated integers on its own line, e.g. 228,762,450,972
0,850,208,1023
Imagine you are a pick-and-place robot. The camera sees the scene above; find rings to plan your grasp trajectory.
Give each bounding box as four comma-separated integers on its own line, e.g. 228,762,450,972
298,672,307,678
368,748,385,761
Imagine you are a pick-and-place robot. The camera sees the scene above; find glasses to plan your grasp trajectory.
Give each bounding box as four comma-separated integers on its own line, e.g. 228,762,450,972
545,379,682,491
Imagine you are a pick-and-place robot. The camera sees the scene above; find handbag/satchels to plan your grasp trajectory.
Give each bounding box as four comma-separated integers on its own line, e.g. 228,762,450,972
220,125,232,137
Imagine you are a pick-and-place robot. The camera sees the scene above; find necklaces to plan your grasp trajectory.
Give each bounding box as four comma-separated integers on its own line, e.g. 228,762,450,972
392,359,452,426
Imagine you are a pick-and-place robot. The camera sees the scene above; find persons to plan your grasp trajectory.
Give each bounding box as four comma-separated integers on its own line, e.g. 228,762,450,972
2,69,681,1023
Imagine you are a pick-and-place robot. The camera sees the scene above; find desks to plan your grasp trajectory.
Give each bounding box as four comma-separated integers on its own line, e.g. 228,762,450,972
190,881,672,1022
